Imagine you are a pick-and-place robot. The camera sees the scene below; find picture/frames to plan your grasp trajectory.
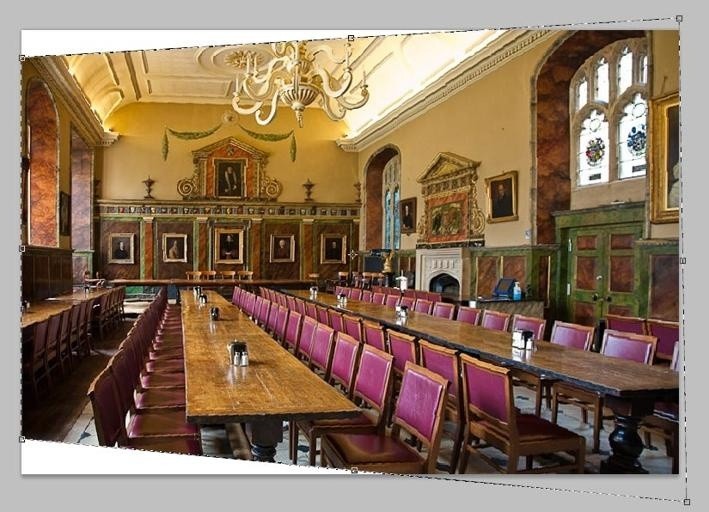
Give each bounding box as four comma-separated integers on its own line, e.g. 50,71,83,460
212,156,248,199
270,234,295,263
162,233,187,263
215,228,244,265
108,232,134,265
320,233,347,264
647,89,680,223
483,170,519,223
399,197,416,234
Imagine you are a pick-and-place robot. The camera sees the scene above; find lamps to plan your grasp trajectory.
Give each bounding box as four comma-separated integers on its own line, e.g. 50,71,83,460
232,42,370,128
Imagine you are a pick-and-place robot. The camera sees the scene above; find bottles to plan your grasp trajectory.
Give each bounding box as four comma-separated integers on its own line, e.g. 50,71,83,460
337,294,347,302
519,335,535,351
512,282,521,300
309,287,318,295
397,308,407,317
525,282,533,298
233,350,249,367
192,286,219,321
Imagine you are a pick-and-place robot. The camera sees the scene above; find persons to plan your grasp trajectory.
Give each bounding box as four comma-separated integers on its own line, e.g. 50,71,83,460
114,240,128,258
492,184,514,217
275,239,290,258
326,241,341,259
403,204,413,230
168,239,182,259
61,199,69,233
221,235,238,259
221,165,240,196
